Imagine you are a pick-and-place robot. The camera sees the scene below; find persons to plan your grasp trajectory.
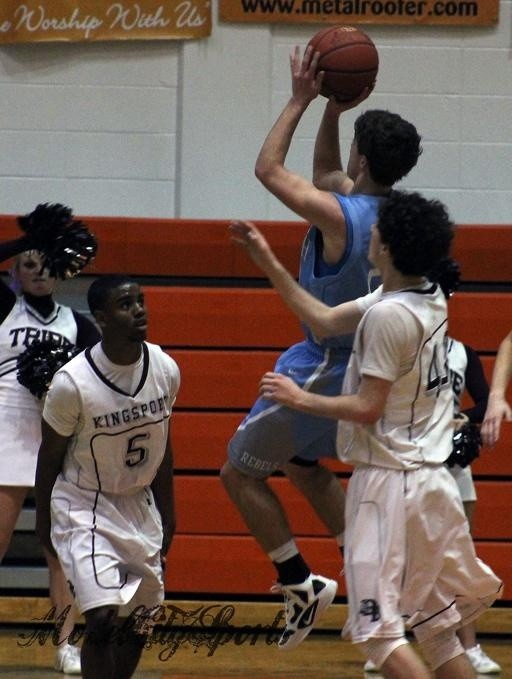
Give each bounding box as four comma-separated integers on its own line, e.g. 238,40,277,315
1,203,104,674
220,43,424,651
35,274,181,678
482,321,511,450
227,192,478,679
364,252,506,675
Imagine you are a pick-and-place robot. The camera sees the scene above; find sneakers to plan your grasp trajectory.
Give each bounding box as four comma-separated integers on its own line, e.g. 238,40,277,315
273,573,339,652
362,660,378,674
466,641,502,675
55,642,82,673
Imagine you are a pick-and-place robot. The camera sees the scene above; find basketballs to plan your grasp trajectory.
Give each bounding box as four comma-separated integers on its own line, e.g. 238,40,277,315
305,24,379,103
